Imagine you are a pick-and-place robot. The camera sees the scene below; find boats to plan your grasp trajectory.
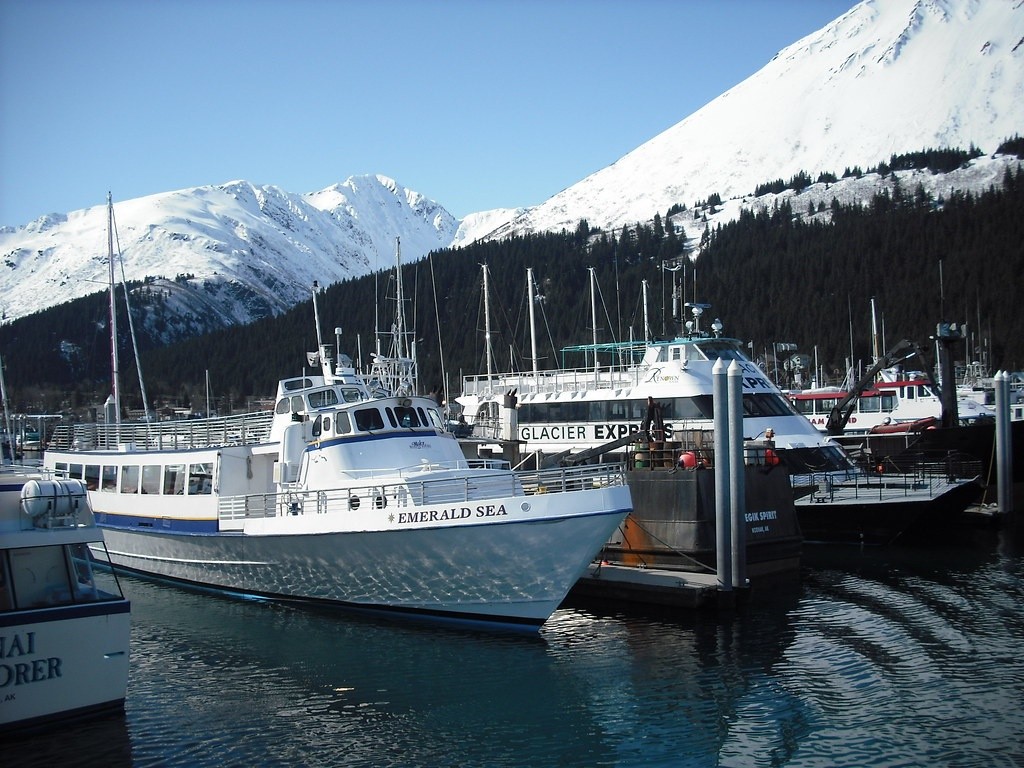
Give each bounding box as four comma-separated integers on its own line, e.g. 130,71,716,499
452,253,1024,490
34,189,634,639
1,351,252,467
0,460,133,736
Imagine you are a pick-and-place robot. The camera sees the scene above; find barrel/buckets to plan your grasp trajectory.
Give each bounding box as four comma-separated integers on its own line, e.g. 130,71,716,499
635,441,649,468
680,451,695,467
650,442,664,467
664,442,682,468
